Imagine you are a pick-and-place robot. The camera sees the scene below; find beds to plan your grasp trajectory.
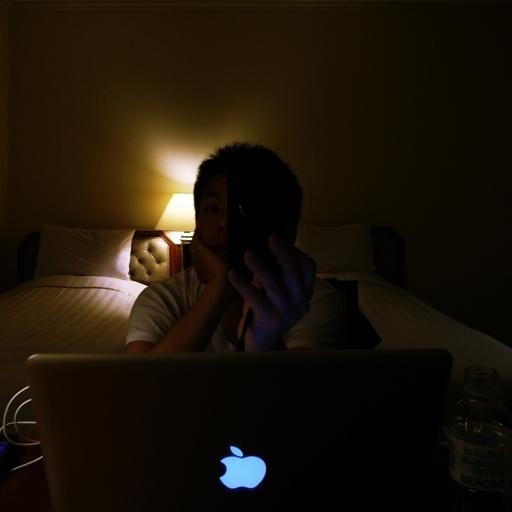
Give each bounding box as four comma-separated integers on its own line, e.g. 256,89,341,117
3,225,509,447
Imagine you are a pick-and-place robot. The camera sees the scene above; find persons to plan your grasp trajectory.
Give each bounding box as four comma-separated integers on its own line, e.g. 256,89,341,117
124,141,343,351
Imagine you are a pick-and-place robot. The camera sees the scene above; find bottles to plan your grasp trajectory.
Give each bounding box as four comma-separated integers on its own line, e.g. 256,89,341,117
445,365,512,512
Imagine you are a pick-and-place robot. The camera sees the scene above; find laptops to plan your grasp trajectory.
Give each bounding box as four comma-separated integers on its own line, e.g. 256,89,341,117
26,348,452,512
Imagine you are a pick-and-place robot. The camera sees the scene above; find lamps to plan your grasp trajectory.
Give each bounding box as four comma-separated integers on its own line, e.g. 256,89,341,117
155,193,198,234
